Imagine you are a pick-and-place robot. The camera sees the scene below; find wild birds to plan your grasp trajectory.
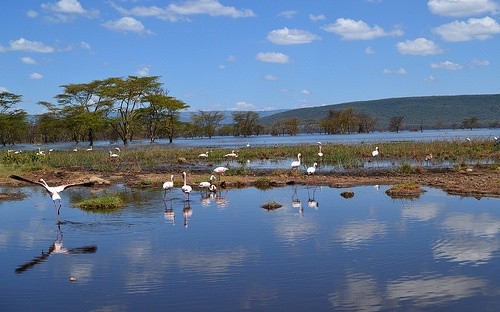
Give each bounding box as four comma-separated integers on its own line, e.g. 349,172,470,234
115,148,121,153
72,146,78,152
289,152,302,176
288,186,304,216
213,166,230,183
180,171,193,202
246,142,250,148
198,174,218,195
466,136,471,143
47,148,54,154
316,142,325,162
493,136,500,142
198,151,209,158
224,149,238,160
182,201,193,230
307,185,320,211
423,151,433,166
108,150,119,159
306,162,318,176
86,145,93,153
373,184,381,190
7,149,24,155
200,190,229,210
163,200,176,227
35,147,45,156
371,146,380,168
8,173,98,224
161,174,174,200
